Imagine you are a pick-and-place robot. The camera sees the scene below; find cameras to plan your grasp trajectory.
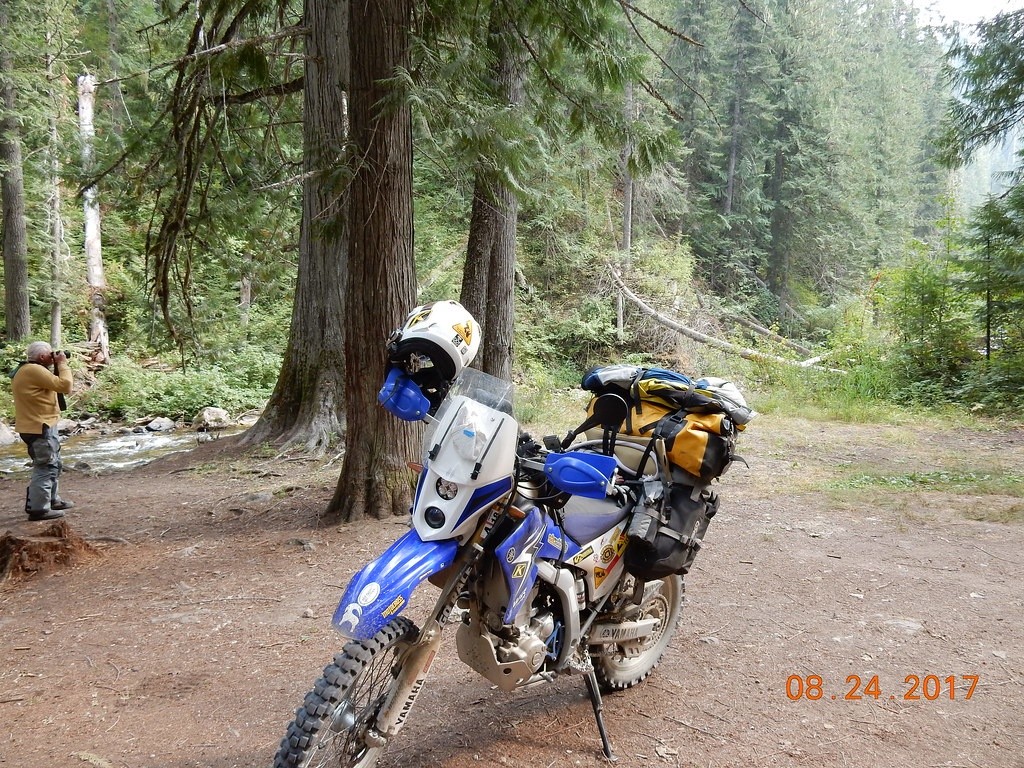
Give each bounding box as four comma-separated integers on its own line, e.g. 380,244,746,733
51,350,70,367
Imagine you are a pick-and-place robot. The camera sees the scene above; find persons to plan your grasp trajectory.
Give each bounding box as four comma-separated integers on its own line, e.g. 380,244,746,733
11,341,74,520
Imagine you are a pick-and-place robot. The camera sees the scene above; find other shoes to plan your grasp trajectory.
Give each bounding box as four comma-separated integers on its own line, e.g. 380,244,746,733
51,499,74,509
28,508,65,521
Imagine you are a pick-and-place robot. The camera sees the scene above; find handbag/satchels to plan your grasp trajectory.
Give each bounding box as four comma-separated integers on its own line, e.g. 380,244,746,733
623,481,721,581
579,366,752,480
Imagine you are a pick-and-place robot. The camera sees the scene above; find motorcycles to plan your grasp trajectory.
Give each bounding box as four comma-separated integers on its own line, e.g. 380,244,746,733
271,364,751,768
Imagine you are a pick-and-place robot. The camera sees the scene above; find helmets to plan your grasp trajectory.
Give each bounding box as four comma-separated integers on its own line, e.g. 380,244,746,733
384,299,483,397
